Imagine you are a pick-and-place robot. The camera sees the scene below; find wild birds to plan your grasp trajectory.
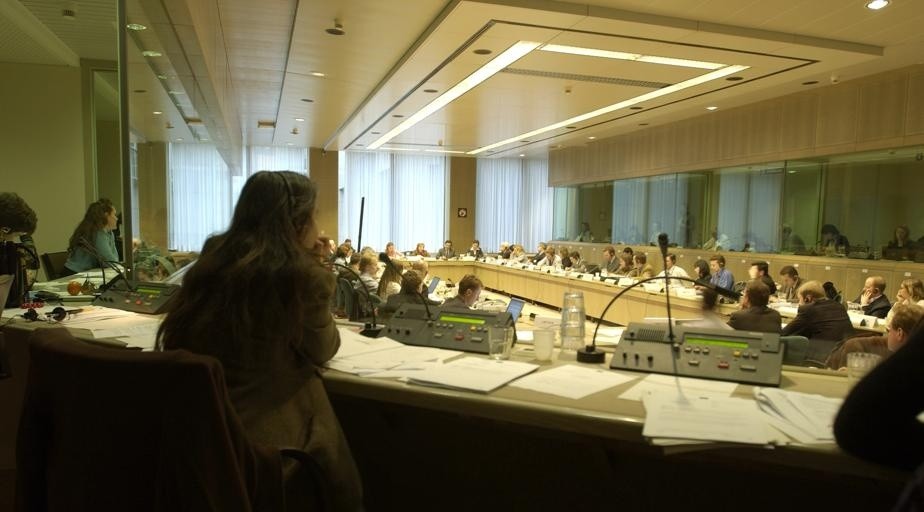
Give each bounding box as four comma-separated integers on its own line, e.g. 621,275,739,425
505,297,527,322
427,275,441,294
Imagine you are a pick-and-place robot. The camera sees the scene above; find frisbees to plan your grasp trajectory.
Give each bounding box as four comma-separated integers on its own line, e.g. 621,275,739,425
805,299,810,303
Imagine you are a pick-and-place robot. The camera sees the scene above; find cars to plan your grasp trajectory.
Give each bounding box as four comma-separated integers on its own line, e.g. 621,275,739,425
487,324,514,362
559,291,587,355
778,292,787,303
459,253,608,279
864,314,877,327
846,351,882,396
531,328,556,362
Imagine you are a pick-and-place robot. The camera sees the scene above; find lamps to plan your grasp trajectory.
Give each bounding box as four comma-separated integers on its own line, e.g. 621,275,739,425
15,326,284,512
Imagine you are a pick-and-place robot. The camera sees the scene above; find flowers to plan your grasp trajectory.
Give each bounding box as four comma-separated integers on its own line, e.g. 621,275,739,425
378,251,431,317
656,231,681,341
68,234,134,292
322,261,388,338
576,274,744,363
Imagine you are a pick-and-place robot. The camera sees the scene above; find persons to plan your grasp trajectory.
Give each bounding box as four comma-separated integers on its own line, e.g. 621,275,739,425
576,221,594,241
498,241,586,272
603,246,735,292
648,220,663,244
832,328,922,512
61,198,121,275
0,192,37,244
820,224,850,246
783,225,805,246
727,260,923,349
888,224,915,249
319,236,484,309
704,224,730,251
172,172,365,508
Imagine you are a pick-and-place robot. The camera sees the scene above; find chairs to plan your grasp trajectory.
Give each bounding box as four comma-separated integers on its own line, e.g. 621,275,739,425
15,326,284,512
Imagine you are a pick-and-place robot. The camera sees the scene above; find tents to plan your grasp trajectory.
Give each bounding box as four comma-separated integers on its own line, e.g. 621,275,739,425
717,256,725,268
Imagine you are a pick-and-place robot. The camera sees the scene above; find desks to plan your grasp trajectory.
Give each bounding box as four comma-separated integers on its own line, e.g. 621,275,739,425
5,235,921,485
